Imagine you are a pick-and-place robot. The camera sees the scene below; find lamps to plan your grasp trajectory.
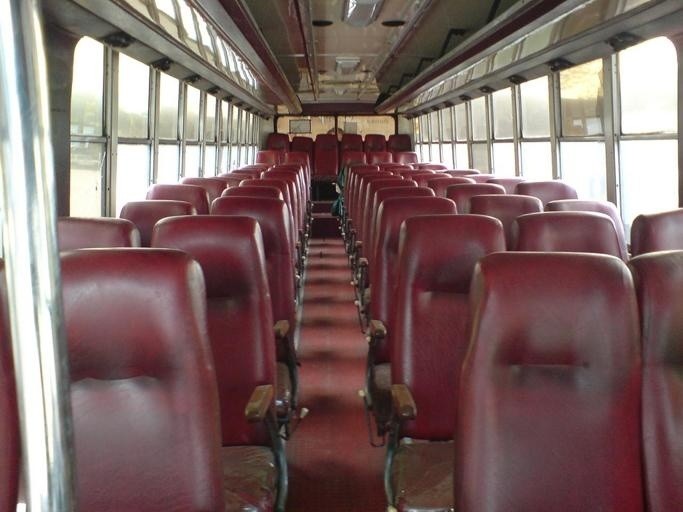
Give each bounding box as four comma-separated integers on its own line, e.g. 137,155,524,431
333,56,360,76
341,0,383,28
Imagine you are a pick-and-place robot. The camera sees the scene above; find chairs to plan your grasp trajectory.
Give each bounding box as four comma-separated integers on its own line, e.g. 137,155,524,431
446,182,506,215
434,168,447,173
340,133,363,167
514,181,579,209
426,177,476,198
221,185,285,203
395,151,418,165
59,245,224,512
374,162,402,171
369,151,392,164
386,133,412,163
291,136,314,174
381,165,414,172
359,195,458,448
119,201,198,247
267,132,290,163
354,185,435,335
544,199,629,264
209,176,242,188
383,211,508,512
464,193,544,251
209,195,309,441
285,178,299,243
350,175,404,288
407,162,431,169
486,177,527,195
314,134,339,176
391,168,436,180
56,215,142,255
339,164,381,241
284,151,311,177
267,167,303,190
363,133,387,164
419,164,447,171
231,169,259,175
412,172,453,187
239,178,296,256
145,183,212,216
273,163,303,177
349,170,394,269
182,176,228,208
444,169,480,177
351,178,418,306
259,169,303,232
150,213,290,512
343,169,362,254
509,210,624,260
630,207,683,257
342,150,367,167
463,174,496,184
255,150,281,168
451,250,646,512
218,174,254,180
626,249,683,512
